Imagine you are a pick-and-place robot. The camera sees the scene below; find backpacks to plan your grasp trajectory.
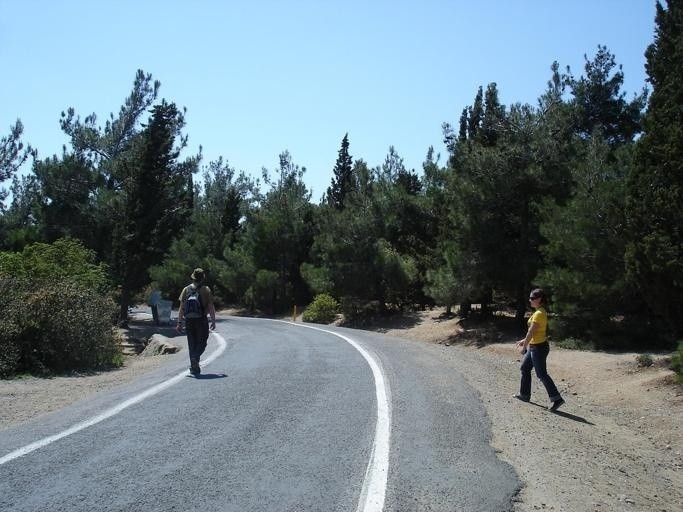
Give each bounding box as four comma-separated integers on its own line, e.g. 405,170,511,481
184,284,204,319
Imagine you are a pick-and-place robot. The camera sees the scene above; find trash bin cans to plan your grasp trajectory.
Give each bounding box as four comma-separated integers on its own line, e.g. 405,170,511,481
157,301,172,327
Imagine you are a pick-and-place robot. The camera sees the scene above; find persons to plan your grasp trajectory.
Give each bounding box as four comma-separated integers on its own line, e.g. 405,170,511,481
513,290,565,413
175,267,216,377
147,287,161,324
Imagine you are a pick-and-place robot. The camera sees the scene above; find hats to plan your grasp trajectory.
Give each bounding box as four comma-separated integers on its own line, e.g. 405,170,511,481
191,268,205,281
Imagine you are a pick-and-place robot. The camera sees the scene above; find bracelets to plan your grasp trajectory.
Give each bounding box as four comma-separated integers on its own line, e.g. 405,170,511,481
177,321,181,324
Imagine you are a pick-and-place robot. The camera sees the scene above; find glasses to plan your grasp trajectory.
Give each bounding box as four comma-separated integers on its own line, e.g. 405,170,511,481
530,297,537,300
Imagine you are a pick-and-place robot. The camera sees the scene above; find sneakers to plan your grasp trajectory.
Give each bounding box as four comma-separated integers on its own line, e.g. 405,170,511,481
551,398,564,410
